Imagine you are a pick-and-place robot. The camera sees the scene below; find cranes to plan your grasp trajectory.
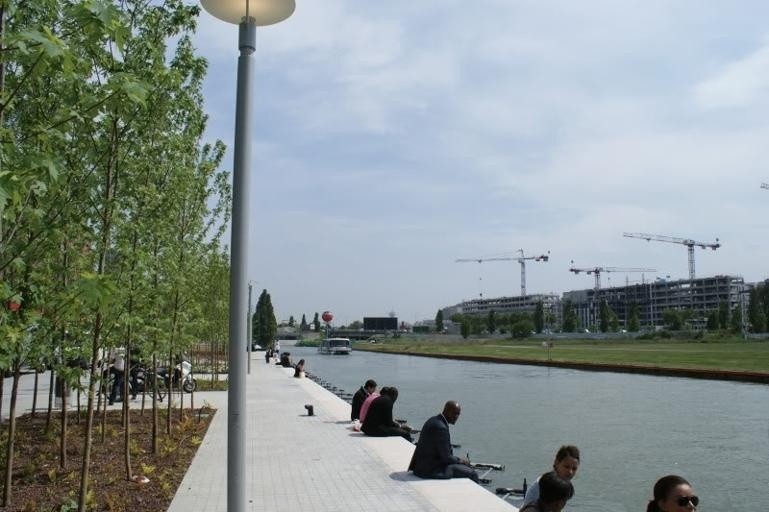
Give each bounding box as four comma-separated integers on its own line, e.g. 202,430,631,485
623,230,720,281
455,246,550,297
568,261,658,290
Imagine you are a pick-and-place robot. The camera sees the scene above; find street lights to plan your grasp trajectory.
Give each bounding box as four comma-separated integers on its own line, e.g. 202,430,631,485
246,279,260,375
198,0,293,511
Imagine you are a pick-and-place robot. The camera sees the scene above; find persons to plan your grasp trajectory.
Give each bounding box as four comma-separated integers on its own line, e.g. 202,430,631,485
360,387,413,441
406,401,479,483
350,379,377,420
519,445,580,507
264,342,305,378
647,476,698,512
518,473,574,512
124,341,146,400
359,386,389,427
99,342,126,403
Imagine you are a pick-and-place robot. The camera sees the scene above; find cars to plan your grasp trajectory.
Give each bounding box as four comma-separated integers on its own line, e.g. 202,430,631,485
1,330,127,376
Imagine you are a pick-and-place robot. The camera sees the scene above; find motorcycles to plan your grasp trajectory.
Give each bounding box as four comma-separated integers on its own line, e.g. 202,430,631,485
140,360,197,392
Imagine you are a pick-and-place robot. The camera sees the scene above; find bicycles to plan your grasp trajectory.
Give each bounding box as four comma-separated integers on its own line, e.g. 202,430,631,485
100,364,166,401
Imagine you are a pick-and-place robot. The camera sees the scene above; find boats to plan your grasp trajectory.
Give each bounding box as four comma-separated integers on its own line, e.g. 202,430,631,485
318,336,353,356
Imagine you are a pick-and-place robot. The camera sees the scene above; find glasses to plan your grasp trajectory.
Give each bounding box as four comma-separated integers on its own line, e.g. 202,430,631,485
668,495,699,507
